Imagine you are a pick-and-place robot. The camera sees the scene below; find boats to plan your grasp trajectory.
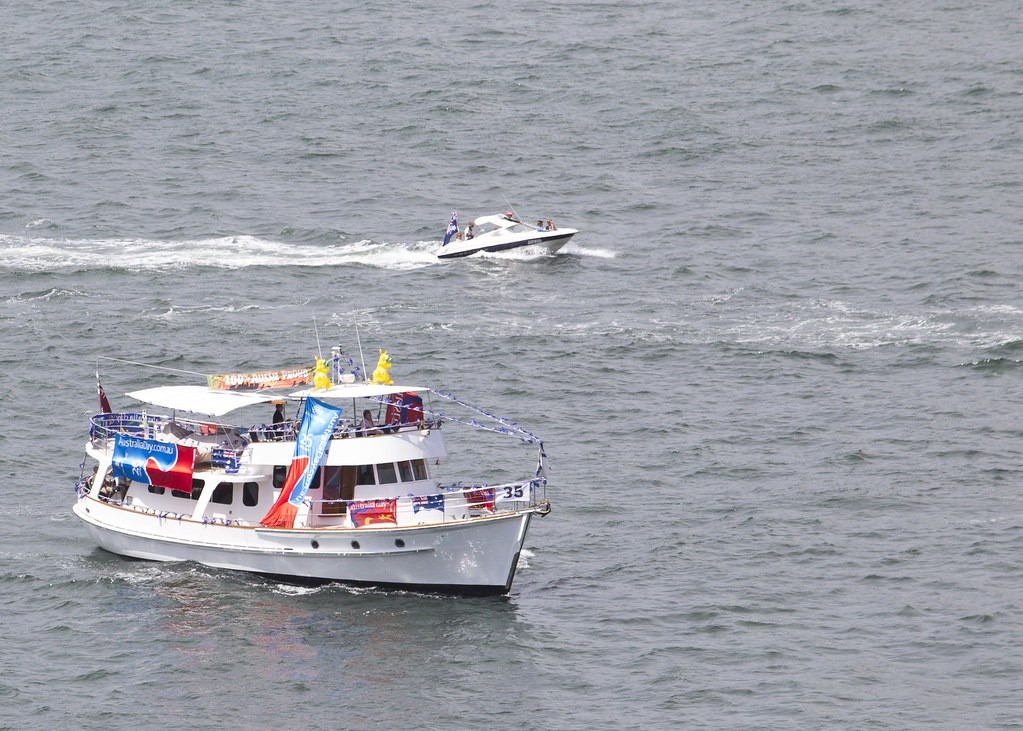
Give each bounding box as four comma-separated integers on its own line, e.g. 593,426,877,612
72,348,552,595
437,214,580,258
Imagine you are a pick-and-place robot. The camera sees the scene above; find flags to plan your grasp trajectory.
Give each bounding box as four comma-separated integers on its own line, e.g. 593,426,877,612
346,496,399,527
96,381,112,413
411,494,444,515
442,207,459,247
462,486,498,513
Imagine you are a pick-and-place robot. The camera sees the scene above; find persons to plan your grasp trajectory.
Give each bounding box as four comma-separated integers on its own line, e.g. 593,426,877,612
361,410,385,436
272,402,291,442
464,222,474,241
93,466,126,499
537,218,556,232
456,233,462,242
